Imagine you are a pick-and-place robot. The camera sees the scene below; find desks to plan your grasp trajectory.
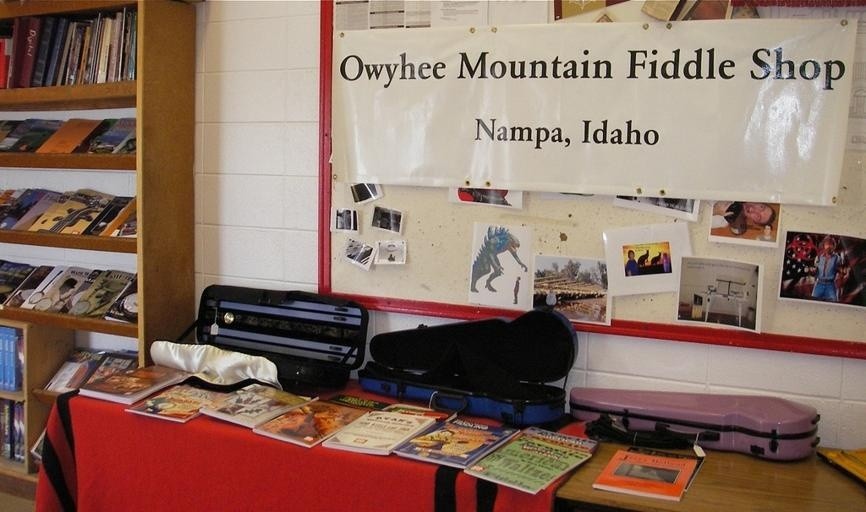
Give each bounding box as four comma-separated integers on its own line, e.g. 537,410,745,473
39,348,866,512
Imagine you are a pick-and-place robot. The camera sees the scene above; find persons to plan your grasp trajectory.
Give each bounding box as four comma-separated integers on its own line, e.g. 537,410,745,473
808,236,850,302
713,203,775,234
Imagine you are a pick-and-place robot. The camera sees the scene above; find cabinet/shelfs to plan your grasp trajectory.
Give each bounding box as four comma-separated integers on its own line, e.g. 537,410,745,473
0,1,195,486
